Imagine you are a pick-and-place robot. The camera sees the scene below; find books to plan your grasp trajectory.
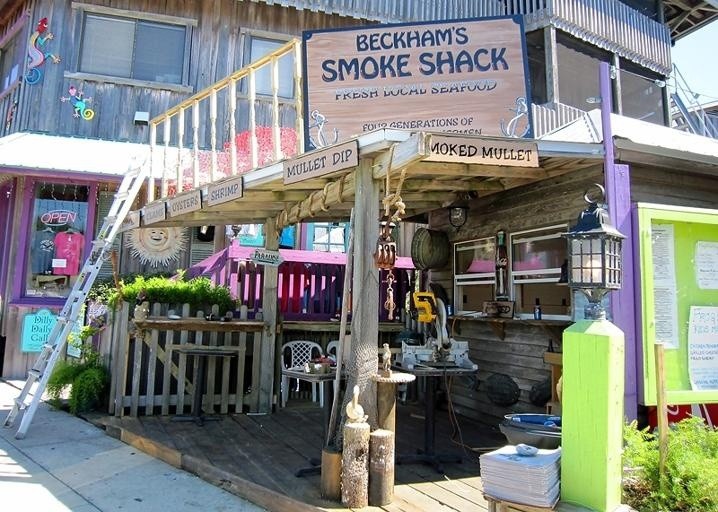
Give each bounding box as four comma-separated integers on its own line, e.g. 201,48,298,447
480,445,560,508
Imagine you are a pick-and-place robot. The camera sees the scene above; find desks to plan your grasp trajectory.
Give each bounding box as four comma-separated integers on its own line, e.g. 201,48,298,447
394,359,477,476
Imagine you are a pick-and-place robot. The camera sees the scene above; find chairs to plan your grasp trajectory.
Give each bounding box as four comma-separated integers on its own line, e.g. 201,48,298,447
280,335,347,413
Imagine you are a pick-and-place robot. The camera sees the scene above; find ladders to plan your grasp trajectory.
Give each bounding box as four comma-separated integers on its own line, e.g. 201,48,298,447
3,144,150,439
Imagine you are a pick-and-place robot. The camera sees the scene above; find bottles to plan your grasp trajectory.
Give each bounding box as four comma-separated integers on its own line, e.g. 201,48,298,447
495,231,509,297
534,298,541,321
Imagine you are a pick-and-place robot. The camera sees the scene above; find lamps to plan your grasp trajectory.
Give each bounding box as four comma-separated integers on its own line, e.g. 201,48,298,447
375,213,398,268
447,189,477,232
